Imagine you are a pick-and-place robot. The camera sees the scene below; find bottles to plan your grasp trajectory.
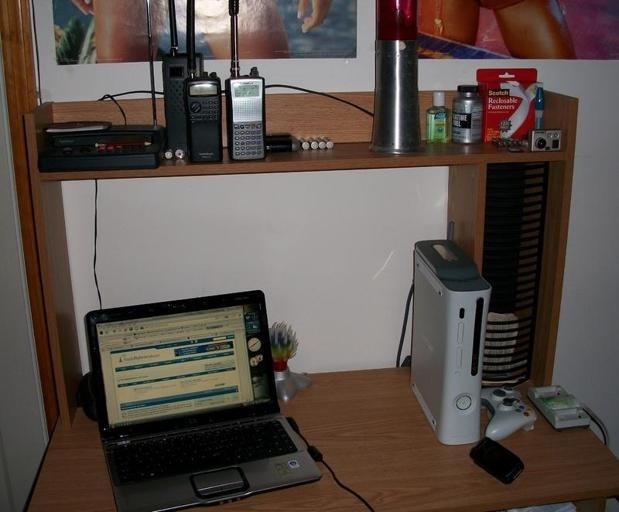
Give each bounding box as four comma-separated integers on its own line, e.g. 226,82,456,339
452,85,483,144
426,91,452,143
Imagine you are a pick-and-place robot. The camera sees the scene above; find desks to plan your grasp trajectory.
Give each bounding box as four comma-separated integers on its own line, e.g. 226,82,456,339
23,89,619,512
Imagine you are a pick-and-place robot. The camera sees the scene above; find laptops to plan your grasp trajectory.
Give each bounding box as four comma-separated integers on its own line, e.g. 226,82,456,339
83,288,323,512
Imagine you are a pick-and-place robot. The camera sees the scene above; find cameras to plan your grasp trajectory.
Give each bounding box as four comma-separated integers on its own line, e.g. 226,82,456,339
529,128,562,152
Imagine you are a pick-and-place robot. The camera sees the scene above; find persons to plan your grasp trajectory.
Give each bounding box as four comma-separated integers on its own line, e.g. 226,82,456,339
70,0,335,63
416,1,572,59
